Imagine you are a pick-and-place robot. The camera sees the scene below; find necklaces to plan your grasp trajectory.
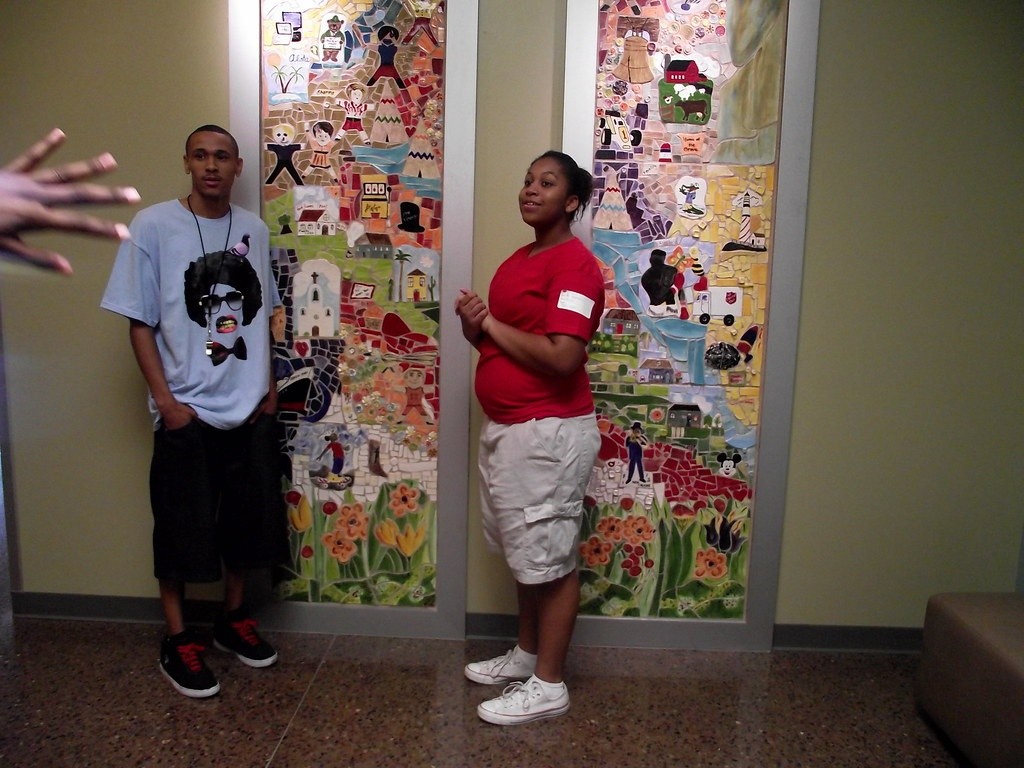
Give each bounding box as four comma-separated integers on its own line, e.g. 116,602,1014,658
186,192,236,360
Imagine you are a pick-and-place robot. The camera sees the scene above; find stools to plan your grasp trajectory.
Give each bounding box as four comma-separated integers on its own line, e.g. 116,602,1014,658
910,589,1023,768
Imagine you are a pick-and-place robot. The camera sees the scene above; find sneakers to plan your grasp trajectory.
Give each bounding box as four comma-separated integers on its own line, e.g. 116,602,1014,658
463,645,534,685
213,609,277,667
478,680,570,726
159,631,221,696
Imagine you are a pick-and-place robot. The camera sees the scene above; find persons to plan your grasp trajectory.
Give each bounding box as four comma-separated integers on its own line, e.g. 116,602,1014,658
454,149,606,726
0,125,144,752
97,123,288,700
